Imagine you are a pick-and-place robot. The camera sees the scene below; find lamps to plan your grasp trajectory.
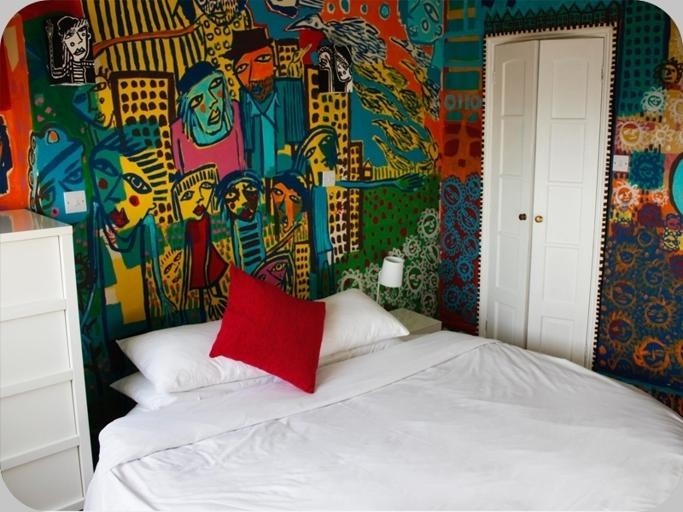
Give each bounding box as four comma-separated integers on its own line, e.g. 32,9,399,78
373,255,406,306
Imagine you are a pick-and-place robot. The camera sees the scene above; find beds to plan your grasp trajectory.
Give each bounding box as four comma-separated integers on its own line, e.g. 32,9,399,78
93,333,683,509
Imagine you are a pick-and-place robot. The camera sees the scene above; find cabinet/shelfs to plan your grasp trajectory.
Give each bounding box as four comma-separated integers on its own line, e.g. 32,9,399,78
0,203,95,509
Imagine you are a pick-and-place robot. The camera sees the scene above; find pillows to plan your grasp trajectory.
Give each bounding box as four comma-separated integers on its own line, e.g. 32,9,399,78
111,339,405,409
210,262,326,394
116,287,412,391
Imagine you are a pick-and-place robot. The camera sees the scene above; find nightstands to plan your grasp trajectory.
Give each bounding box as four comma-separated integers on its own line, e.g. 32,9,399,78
388,307,444,341
